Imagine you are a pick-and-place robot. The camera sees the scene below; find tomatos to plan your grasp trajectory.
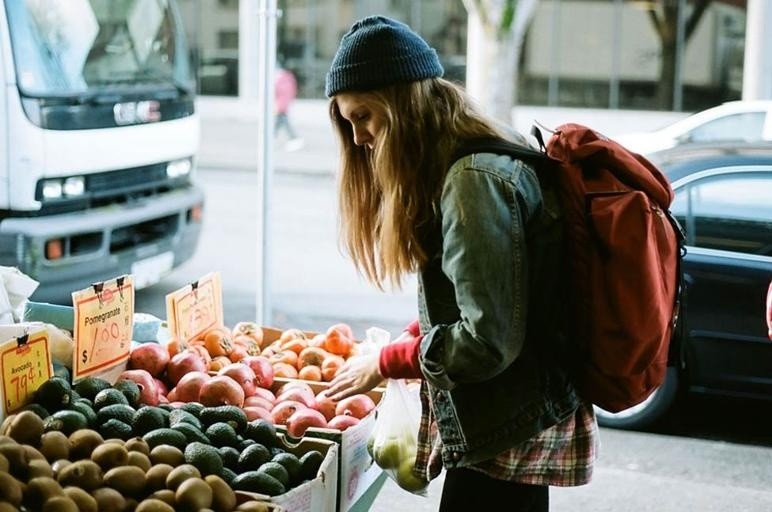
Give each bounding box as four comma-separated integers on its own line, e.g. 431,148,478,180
191,321,354,382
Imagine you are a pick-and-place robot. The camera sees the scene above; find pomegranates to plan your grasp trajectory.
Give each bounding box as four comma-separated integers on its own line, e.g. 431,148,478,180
117,346,375,436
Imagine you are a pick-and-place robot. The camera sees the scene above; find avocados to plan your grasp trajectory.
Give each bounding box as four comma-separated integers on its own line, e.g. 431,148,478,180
21,375,324,495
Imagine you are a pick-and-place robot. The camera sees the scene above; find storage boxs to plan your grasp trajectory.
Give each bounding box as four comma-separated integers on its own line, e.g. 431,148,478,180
235,433,341,512
204,369,390,512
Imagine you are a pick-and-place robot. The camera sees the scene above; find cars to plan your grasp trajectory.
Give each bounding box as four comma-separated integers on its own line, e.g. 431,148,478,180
616,96,772,156
590,156,771,434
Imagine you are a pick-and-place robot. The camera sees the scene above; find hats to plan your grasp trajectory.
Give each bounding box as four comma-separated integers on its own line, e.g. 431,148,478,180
326,16,442,97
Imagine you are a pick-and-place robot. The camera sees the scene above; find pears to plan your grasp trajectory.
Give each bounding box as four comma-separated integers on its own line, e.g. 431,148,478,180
365,433,430,495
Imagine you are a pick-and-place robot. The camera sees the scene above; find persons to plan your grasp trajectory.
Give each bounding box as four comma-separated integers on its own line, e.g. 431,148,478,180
325,14,596,510
274,53,297,140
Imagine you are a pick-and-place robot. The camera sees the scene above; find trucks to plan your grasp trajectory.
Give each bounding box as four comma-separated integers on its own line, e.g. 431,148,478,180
0,1,206,305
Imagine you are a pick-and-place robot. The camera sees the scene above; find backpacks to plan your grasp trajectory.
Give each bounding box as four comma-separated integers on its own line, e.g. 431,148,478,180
447,119,687,414
